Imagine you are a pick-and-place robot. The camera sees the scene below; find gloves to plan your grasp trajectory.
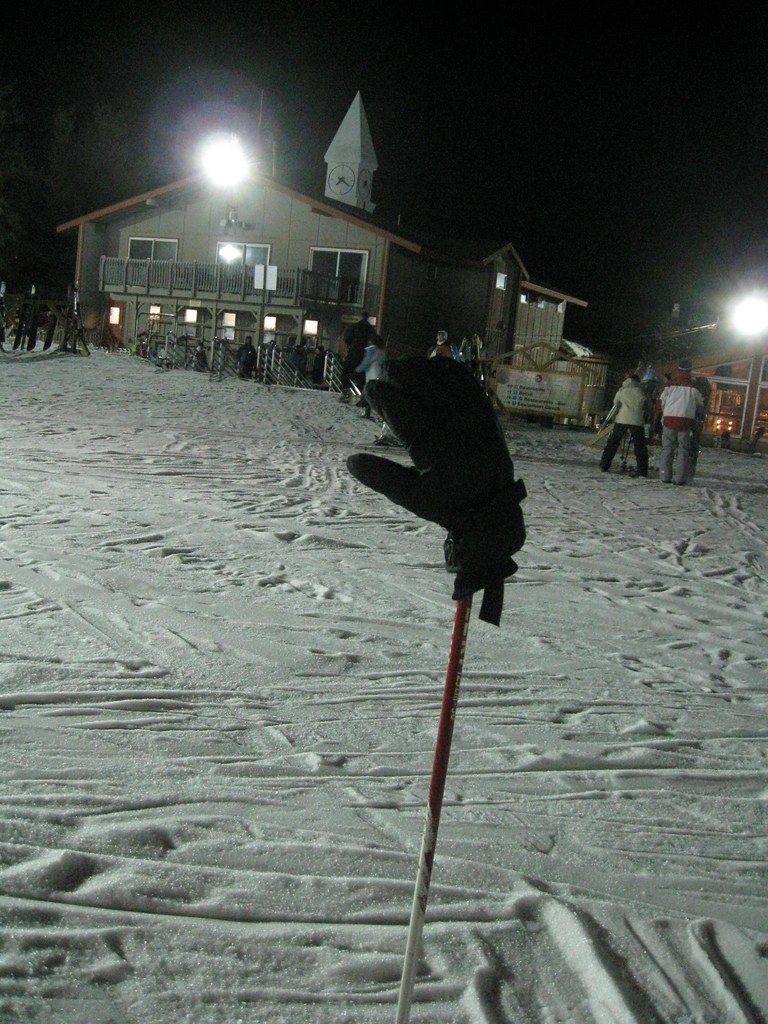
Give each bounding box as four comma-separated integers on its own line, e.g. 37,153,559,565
346,356,526,627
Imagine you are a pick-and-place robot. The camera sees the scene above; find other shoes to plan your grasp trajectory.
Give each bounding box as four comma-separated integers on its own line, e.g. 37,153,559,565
360,413,370,418
601,467,608,472
638,473,648,477
345,394,350,400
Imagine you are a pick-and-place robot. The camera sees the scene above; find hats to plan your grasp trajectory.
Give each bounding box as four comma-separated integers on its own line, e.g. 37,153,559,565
678,361,693,372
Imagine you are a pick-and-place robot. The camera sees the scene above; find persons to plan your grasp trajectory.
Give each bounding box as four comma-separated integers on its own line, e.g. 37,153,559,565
427,330,454,361
310,346,326,384
336,312,376,407
355,334,387,423
598,373,652,480
656,358,712,487
126,338,137,353
234,336,257,380
286,344,307,379
191,342,210,372
